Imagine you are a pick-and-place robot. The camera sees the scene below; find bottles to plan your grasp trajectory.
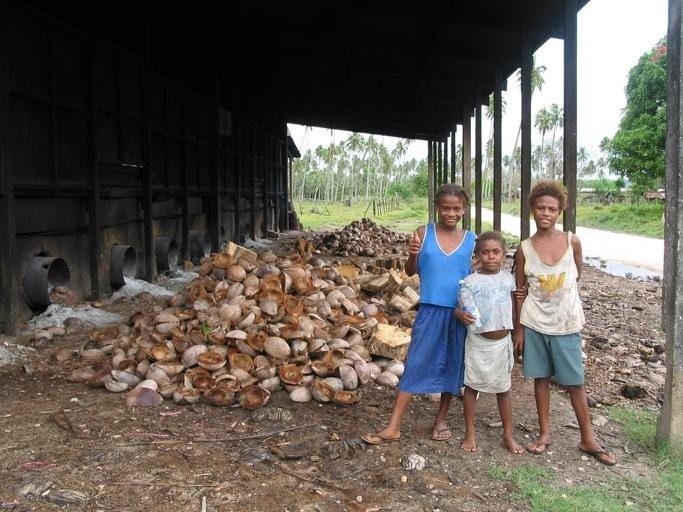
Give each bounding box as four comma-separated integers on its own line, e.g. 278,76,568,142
456,279,483,334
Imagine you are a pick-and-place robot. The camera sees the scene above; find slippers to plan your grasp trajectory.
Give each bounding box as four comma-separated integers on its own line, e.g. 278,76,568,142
578,442,616,465
431,427,453,441
360,430,402,445
525,438,554,454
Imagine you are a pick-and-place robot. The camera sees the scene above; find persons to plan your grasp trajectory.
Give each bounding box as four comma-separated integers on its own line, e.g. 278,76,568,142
513,181,617,467
453,232,529,454
359,185,480,446
